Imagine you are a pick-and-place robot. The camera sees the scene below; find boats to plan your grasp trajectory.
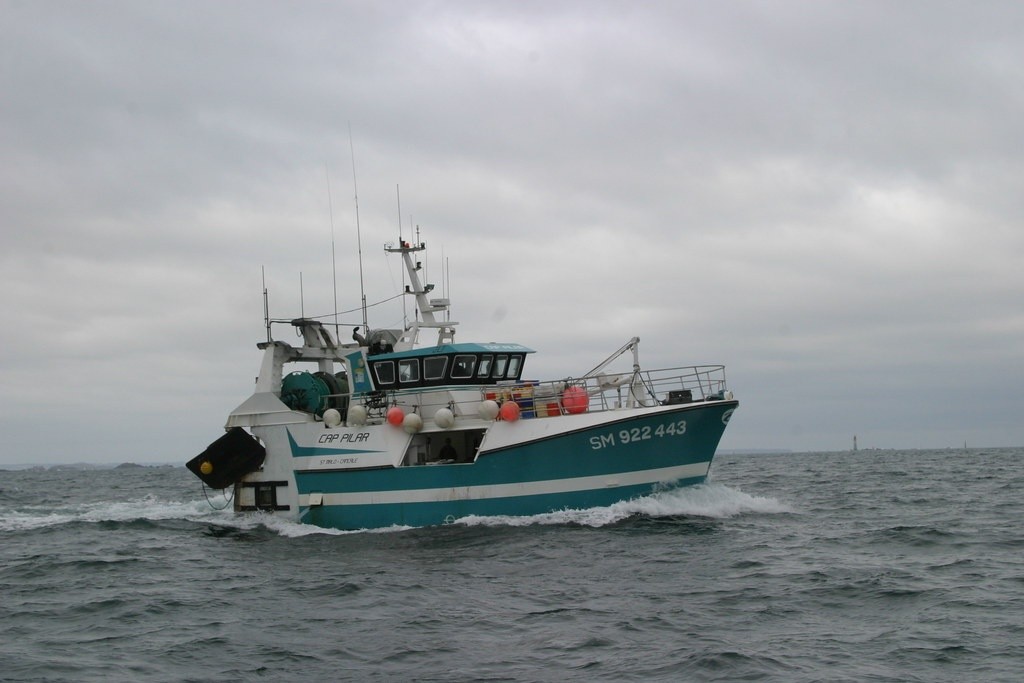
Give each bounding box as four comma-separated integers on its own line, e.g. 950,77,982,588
185,149,739,531
